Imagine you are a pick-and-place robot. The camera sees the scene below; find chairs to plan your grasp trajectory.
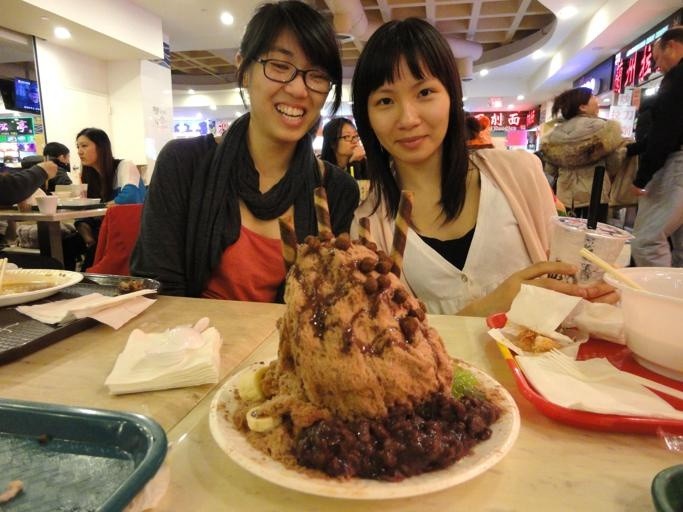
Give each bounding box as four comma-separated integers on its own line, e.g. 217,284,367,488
86,202,143,276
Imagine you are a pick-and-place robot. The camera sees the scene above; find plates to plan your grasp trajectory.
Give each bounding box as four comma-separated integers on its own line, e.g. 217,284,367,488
486,310,683,432
0,398,167,511
209,356,522,501
32,202,108,210
0,272,160,365
1,269,84,308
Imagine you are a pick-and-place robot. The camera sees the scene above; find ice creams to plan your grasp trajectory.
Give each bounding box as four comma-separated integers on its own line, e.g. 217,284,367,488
249,187,498,480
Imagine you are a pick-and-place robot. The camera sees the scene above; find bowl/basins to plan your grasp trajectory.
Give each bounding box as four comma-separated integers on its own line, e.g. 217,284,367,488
602,267,683,381
37,196,57,214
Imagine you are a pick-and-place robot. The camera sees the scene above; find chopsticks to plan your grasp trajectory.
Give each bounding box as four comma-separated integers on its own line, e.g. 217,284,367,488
44,153,49,192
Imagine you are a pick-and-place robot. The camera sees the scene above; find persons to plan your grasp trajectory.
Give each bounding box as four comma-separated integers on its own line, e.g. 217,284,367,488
127,0,360,304
630,28,683,267
315,118,378,181
21,143,71,192
461,116,496,156
538,85,628,224
0,159,56,205
350,16,620,317
63,128,146,273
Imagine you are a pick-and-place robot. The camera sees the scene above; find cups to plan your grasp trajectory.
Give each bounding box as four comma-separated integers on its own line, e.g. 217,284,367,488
547,214,635,292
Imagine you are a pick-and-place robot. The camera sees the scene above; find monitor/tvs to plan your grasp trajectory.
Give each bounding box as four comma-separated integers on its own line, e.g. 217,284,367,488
0,77,40,115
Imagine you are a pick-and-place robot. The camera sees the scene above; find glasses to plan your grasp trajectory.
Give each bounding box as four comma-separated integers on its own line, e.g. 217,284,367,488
249,55,335,96
340,135,361,143
